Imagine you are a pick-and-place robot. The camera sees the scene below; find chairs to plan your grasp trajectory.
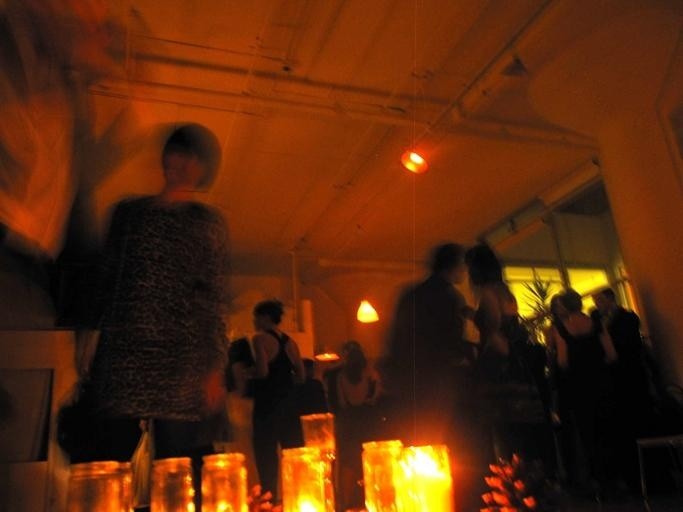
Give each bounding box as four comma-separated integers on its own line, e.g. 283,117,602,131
635,435,683,512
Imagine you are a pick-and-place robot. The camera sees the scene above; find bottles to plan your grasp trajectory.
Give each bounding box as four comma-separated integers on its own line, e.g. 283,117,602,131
65,414,451,512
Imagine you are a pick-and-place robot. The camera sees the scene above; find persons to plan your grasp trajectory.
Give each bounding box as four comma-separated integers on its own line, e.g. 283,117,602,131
231,299,380,504
379,243,642,507
45,74,231,512
